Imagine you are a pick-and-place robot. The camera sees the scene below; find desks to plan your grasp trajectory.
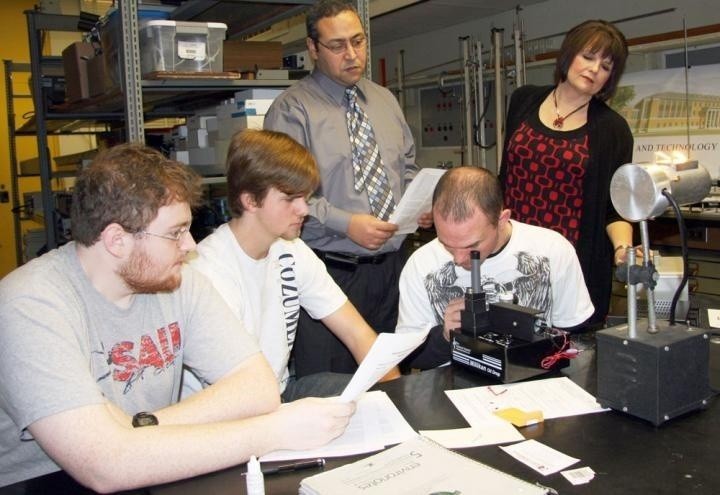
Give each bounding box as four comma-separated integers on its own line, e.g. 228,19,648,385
1,331,720,493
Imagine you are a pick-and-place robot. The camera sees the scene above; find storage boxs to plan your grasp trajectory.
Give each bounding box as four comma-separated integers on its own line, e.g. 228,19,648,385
139,18,229,77
61,35,102,104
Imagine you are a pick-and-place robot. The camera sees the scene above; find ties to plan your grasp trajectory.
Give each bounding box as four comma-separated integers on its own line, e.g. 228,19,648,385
344,85,397,222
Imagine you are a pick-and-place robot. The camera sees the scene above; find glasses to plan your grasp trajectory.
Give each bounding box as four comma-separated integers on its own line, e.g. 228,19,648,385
122,225,191,248
317,38,368,54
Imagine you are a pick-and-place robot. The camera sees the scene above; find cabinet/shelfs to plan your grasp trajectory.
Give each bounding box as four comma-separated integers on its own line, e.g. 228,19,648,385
2,1,372,266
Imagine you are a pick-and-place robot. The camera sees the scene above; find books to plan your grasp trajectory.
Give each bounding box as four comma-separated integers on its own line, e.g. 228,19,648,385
294,433,551,495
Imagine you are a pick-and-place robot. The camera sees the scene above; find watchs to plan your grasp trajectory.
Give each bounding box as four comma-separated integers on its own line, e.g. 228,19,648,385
130,411,159,428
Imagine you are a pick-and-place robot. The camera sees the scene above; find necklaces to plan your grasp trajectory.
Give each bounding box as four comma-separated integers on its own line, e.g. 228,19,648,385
553,86,592,129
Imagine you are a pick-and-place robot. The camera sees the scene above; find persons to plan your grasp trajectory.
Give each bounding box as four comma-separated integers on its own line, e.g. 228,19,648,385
175,127,401,403
0,139,359,495
393,164,597,376
499,18,633,336
262,0,434,382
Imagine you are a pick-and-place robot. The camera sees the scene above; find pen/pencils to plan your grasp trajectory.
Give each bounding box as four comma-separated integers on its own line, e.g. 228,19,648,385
240,459,327,478
